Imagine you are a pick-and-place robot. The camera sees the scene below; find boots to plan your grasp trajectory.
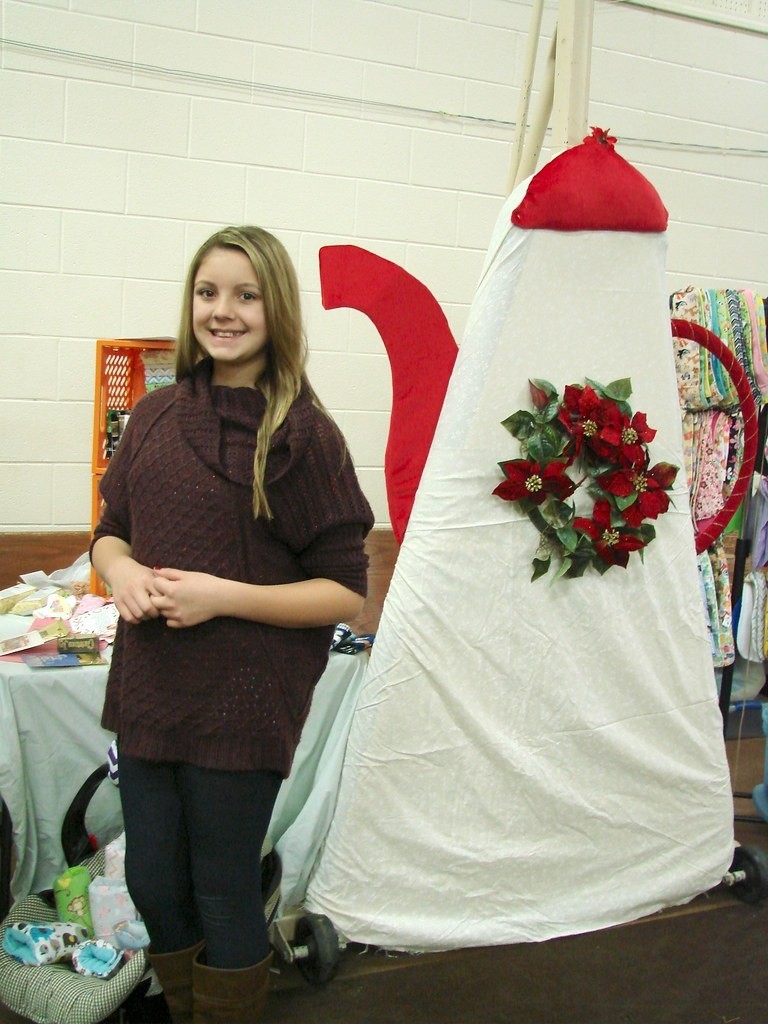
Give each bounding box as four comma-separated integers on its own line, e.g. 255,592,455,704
144,940,205,1024
193,943,274,1024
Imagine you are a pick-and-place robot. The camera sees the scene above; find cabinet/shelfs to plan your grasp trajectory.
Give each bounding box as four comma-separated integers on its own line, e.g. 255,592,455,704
90,339,178,597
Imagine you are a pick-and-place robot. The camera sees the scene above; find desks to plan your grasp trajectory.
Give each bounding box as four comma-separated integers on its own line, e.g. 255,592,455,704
0,554,368,918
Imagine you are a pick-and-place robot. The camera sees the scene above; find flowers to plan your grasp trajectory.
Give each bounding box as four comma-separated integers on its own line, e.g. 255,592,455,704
492,372,682,579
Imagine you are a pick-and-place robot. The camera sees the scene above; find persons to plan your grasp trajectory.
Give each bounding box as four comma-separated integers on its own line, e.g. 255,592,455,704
90,226,374,1024
